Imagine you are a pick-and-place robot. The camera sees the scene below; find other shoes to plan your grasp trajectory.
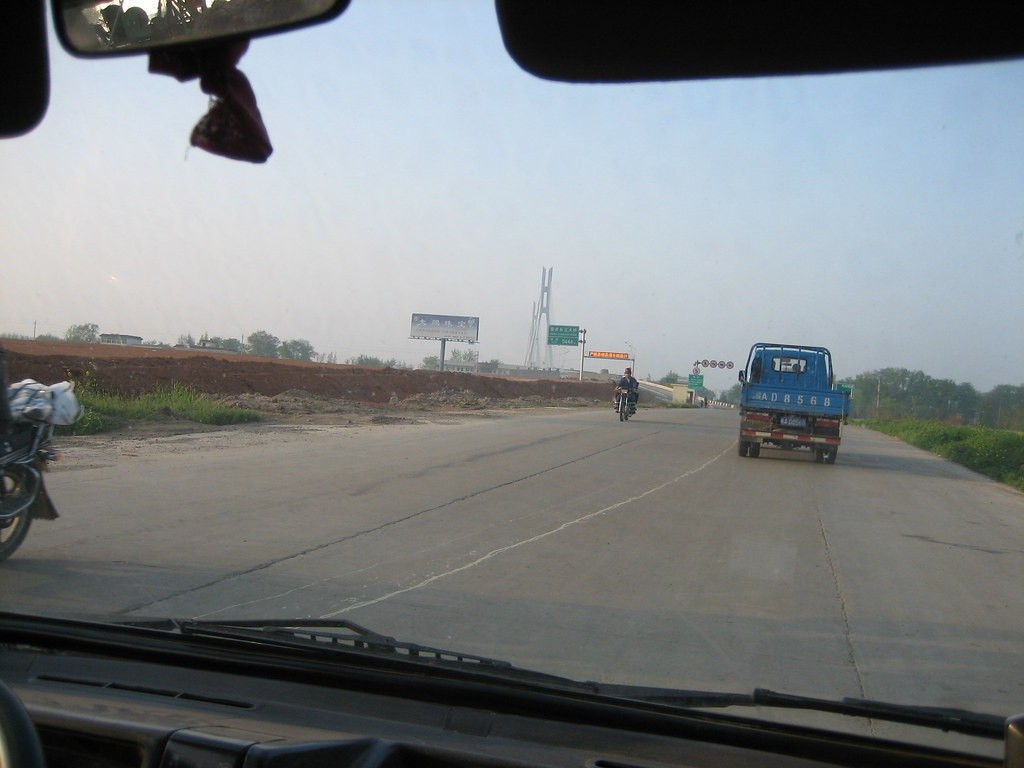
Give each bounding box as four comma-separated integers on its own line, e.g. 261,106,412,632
615,407,618,413
630,407,636,410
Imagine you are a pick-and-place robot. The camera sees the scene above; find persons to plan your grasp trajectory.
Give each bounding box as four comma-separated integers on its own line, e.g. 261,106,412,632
0,344,13,526
93,5,148,44
704,396,707,408
614,368,639,414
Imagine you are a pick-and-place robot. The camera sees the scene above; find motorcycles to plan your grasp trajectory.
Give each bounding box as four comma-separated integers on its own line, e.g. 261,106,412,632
613,387,638,422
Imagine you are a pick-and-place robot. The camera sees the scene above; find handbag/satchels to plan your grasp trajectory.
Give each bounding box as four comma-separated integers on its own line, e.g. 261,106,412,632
6,378,79,425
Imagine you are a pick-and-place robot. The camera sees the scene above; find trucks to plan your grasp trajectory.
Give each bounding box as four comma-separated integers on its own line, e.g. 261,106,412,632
737,342,852,464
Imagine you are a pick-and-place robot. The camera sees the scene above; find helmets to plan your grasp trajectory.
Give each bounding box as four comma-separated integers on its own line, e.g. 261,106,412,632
624,368,631,374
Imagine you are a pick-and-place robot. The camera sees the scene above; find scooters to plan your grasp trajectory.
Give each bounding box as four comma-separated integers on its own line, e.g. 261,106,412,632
0,415,61,564
